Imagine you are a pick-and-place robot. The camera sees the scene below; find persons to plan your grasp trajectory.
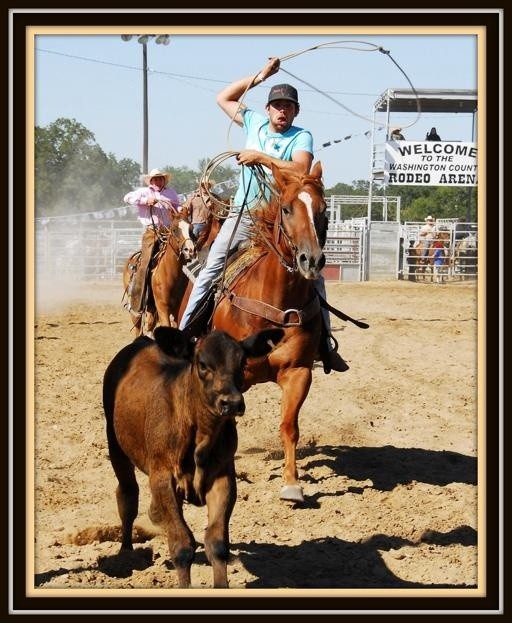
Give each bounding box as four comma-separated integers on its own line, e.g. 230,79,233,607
431,242,445,282
122,168,182,229
399,237,406,278
185,174,223,239
407,239,417,282
179,57,349,372
420,216,437,261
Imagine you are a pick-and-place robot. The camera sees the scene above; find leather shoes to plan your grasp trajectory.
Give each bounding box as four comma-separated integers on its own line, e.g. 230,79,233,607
316,350,349,372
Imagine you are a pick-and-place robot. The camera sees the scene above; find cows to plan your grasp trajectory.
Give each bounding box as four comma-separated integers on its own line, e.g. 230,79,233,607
103,325,285,589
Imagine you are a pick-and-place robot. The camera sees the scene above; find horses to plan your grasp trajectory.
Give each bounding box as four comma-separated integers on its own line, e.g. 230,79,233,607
415,230,477,281
177,160,328,502
123,196,232,341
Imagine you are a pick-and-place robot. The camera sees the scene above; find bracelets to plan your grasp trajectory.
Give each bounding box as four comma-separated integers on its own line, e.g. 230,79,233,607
258,74,265,81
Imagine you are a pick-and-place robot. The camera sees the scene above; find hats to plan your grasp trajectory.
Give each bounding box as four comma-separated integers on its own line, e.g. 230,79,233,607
141,169,171,187
196,176,216,189
424,216,435,222
268,84,298,105
435,243,442,248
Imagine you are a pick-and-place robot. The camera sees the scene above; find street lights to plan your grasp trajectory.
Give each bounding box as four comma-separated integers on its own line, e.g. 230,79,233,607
121,34,170,177
